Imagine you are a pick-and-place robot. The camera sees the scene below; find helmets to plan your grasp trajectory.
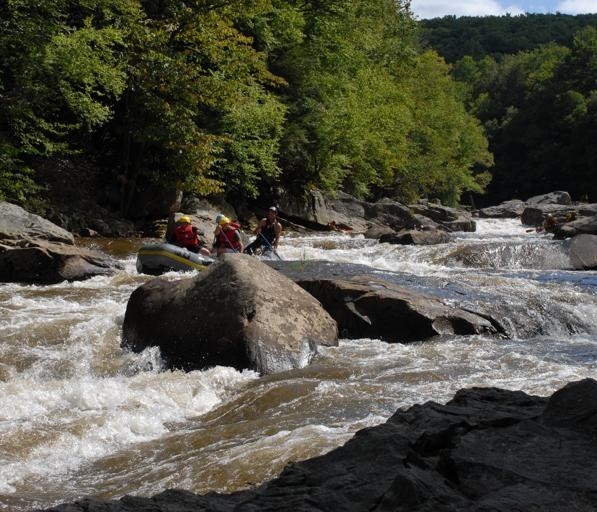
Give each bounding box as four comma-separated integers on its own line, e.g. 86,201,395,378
180,217,190,223
216,215,229,225
268,207,278,214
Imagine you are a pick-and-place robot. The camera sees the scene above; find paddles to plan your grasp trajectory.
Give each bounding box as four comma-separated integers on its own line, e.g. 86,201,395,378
526,228,541,233
535,226,547,232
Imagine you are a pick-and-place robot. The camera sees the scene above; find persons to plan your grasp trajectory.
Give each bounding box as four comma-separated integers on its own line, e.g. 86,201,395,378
242,205,282,260
214,213,241,238
214,217,243,258
174,216,211,257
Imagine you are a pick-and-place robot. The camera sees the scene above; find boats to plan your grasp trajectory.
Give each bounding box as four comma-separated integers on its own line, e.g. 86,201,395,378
134,242,287,277
